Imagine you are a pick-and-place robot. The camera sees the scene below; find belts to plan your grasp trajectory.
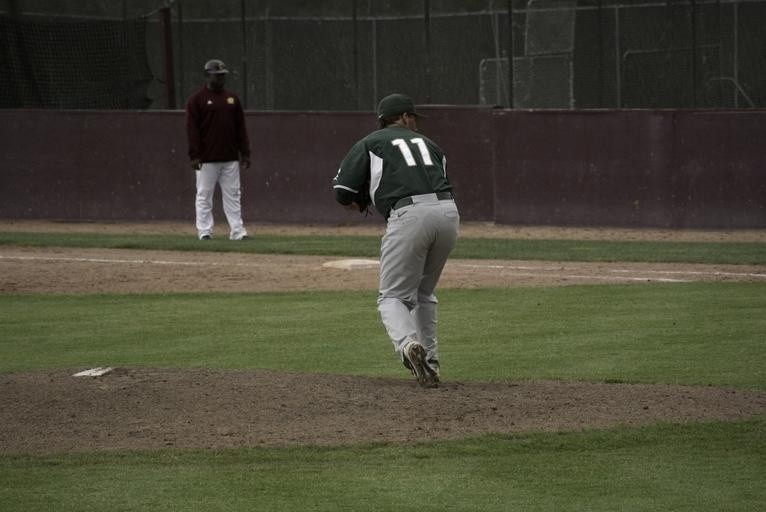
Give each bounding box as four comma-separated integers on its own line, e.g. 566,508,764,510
392,190,453,211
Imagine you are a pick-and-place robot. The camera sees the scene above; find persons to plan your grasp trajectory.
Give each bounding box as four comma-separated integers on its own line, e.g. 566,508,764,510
331,91,462,389
185,58,253,242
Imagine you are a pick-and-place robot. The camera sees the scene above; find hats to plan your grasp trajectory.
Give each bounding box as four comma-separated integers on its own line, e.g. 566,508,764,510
204,59,229,75
376,92,429,120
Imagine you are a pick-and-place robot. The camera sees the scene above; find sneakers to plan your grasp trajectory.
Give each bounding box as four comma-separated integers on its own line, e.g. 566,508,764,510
200,233,211,239
403,340,442,387
240,235,249,239
